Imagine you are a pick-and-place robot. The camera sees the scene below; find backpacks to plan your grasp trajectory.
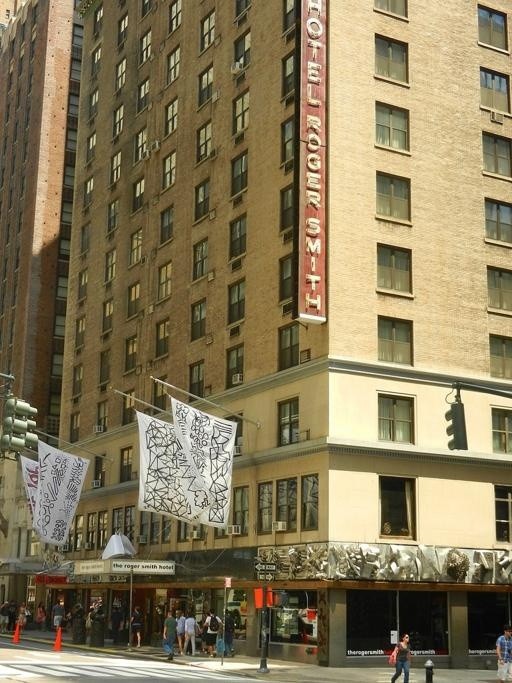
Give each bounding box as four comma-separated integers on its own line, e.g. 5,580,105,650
208,614,219,631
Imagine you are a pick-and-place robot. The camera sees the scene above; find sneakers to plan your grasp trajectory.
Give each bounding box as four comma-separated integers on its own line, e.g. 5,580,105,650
178,646,241,659
167,652,174,661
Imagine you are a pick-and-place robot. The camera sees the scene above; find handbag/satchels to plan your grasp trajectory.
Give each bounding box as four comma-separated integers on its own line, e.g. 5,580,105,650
85,611,92,630
388,644,399,667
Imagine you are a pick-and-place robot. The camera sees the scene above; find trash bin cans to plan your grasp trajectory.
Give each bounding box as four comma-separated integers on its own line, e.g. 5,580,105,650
71,603,104,647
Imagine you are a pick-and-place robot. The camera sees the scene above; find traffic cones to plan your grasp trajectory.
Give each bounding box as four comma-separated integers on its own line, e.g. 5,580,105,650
52,626,64,651
12,620,21,645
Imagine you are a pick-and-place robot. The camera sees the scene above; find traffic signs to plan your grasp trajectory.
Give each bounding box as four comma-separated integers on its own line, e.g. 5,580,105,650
257,573,275,581
255,563,277,571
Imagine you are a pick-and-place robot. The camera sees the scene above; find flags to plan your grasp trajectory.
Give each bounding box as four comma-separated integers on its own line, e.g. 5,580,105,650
33,439,91,548
168,398,238,529
21,455,39,517
135,410,196,526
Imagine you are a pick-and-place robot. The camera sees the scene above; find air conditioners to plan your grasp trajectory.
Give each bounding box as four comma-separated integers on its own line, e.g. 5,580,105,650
84,542,92,549
231,62,242,74
142,149,150,161
136,536,147,544
90,480,101,489
150,140,161,153
232,374,242,385
57,544,69,552
272,521,287,531
233,446,243,456
189,531,201,540
93,425,104,434
226,524,241,535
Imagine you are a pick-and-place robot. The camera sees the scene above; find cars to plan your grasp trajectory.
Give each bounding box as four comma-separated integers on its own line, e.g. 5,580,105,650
183,597,318,643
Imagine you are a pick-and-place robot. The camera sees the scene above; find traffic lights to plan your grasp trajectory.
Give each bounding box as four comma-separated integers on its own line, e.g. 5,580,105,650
274,592,289,607
3,399,30,453
445,403,468,452
25,405,38,446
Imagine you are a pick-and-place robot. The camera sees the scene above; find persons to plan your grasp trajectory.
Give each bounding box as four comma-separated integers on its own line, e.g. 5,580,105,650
0,599,65,634
128,607,141,649
161,609,234,659
495,625,512,683
390,633,410,683
